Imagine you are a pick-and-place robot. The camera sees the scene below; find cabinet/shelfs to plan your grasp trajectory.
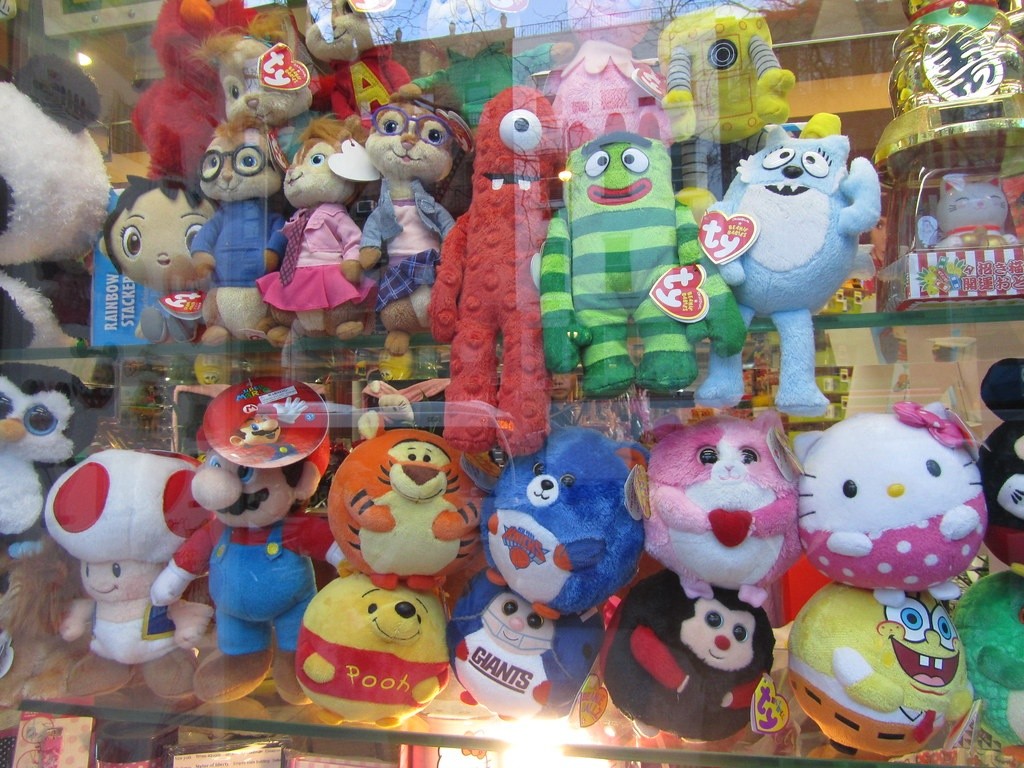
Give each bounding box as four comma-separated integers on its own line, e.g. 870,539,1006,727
0,298,1024,768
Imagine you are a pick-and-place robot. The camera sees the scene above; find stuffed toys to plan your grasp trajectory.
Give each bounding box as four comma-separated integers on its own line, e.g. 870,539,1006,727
327,429,481,590
977,359,1024,574
644,411,801,608
0,0,880,706
45,450,215,699
788,582,973,757
952,569,1024,746
150,426,346,705
600,567,776,745
793,401,988,607
296,557,450,728
446,567,605,721
481,419,651,619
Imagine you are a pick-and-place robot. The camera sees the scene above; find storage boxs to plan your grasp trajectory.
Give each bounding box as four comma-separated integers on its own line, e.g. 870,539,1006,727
87,189,170,345
874,249,1024,313
